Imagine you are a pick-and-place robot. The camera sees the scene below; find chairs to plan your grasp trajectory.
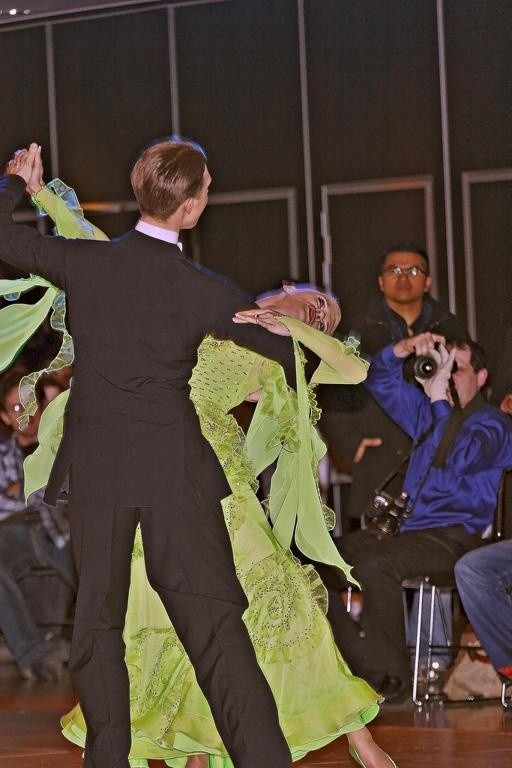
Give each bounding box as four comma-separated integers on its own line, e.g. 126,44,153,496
346,466,506,708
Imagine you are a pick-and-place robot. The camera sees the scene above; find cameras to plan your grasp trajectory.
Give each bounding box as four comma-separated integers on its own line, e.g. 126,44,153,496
405,342,441,381
366,489,410,536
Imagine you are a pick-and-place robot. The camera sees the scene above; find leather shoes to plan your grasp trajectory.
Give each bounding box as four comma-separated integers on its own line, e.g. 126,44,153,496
378,674,411,703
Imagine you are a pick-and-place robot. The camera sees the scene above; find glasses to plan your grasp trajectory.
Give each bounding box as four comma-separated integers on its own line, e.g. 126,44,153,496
381,265,427,276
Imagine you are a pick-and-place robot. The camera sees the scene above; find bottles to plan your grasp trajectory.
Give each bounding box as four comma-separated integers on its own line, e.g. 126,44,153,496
375,489,408,537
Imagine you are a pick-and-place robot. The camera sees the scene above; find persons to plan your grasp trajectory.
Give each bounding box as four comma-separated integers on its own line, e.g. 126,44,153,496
306,237,492,696
451,392,512,735
2,258,80,682
311,327,512,706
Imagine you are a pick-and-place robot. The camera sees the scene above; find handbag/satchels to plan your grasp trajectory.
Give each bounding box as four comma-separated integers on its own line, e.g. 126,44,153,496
443,624,503,702
